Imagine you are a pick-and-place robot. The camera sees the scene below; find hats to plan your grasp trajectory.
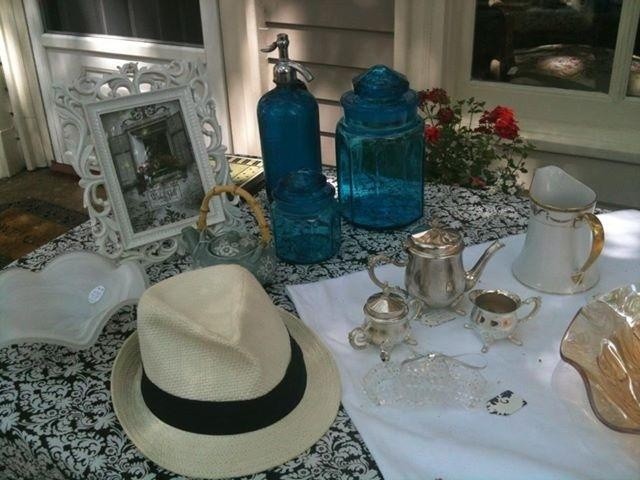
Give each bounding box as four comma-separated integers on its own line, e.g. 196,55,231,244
112,263,343,474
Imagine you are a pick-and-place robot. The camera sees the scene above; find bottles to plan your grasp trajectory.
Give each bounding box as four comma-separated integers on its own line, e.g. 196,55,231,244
336,63,425,230
271,169,338,264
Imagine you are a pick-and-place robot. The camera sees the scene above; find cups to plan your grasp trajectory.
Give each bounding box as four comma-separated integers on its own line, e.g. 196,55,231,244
350,286,424,362
463,288,541,354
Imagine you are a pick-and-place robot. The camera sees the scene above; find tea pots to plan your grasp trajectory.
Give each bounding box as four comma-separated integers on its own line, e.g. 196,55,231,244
509,165,605,295
367,219,505,310
181,186,279,289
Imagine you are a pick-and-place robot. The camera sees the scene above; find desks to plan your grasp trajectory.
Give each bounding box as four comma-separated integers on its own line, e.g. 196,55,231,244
0,154,613,480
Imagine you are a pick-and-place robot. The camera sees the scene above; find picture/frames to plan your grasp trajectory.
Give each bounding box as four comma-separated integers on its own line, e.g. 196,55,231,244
49,55,246,273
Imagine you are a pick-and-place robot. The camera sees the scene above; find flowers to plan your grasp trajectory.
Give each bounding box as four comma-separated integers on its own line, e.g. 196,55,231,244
415,88,536,193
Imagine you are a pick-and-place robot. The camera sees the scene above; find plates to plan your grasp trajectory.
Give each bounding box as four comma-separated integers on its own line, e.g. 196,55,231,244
560,280,640,434
1,252,150,349
